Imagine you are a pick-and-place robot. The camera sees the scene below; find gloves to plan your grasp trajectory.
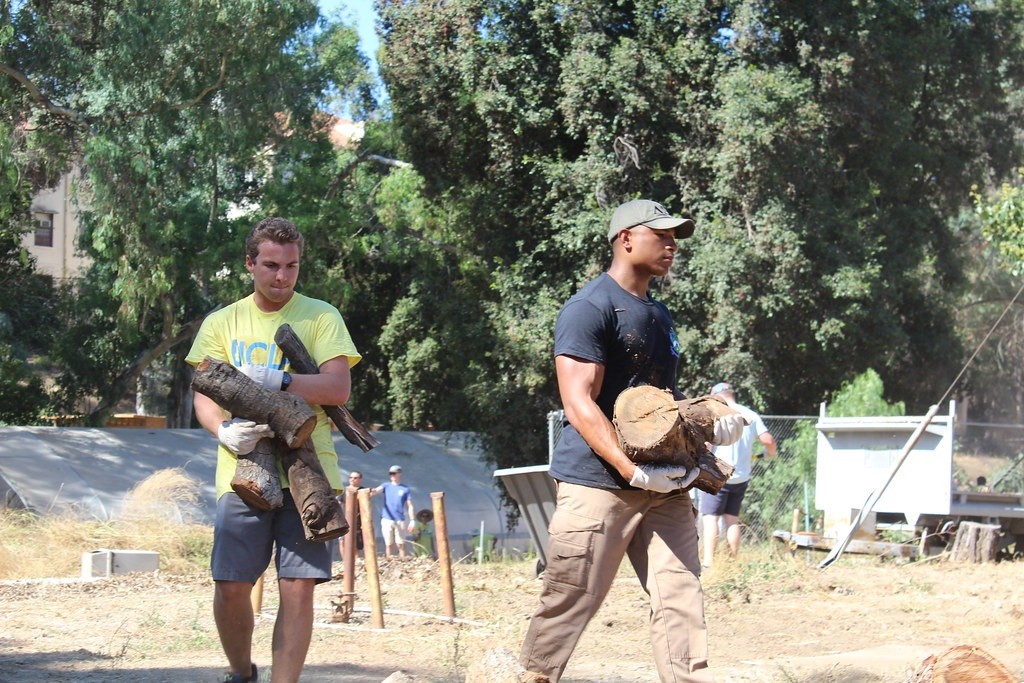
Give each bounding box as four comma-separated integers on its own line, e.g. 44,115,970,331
628,465,700,494
407,520,416,533
235,363,284,393
218,417,276,456
709,414,744,446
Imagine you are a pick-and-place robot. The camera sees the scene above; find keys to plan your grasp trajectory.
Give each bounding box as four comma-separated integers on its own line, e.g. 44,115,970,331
677,476,683,497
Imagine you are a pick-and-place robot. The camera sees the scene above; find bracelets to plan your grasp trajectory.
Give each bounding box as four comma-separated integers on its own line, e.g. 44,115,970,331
410,519,416,526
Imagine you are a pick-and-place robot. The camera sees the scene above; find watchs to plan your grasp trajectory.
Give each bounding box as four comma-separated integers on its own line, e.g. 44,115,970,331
280,372,293,392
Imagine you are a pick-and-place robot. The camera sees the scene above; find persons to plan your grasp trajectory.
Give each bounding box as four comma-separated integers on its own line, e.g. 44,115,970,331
514,199,743,683
336,470,371,566
412,508,438,562
367,466,416,562
185,217,362,683
676,382,777,571
470,529,498,566
974,476,991,494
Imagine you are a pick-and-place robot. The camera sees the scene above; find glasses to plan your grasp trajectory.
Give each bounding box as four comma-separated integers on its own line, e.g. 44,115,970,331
388,471,398,476
422,514,429,517
349,477,359,480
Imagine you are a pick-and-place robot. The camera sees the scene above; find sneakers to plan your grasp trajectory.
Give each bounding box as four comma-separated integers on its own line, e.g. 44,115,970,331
222,662,258,683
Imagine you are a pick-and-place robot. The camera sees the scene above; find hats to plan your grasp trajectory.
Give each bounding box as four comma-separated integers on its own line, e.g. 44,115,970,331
388,465,403,474
711,382,734,396
607,199,697,248
416,508,434,522
